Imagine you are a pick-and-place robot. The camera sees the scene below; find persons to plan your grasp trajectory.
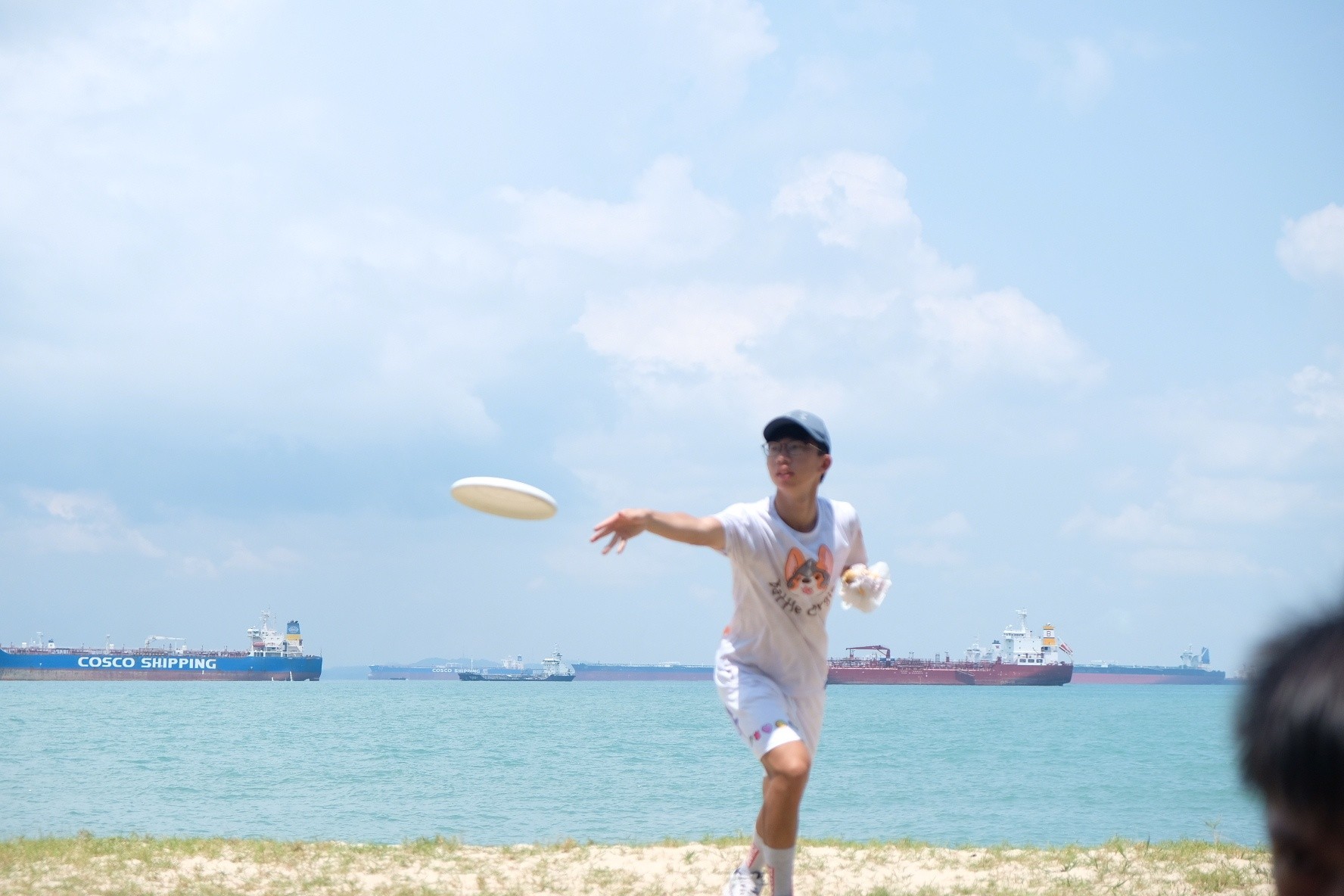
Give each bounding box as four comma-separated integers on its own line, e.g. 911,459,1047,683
1229,613,1344,896
592,411,888,896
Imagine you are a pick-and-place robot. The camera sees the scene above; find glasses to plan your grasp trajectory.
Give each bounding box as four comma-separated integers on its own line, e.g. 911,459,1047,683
762,438,825,458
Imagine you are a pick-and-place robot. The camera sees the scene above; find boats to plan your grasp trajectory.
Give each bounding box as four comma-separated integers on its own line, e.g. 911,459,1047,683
568,661,715,682
0,609,323,683
827,607,1077,688
252,642,265,648
367,663,533,682
458,644,577,682
1067,647,1227,683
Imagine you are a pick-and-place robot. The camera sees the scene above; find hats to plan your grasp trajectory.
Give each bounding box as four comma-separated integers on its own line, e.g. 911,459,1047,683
763,409,832,454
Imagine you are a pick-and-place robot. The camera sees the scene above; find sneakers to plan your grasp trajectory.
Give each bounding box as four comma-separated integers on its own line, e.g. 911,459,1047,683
723,865,765,896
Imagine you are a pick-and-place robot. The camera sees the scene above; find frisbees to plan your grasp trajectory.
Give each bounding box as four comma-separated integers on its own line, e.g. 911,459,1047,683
451,476,558,521
